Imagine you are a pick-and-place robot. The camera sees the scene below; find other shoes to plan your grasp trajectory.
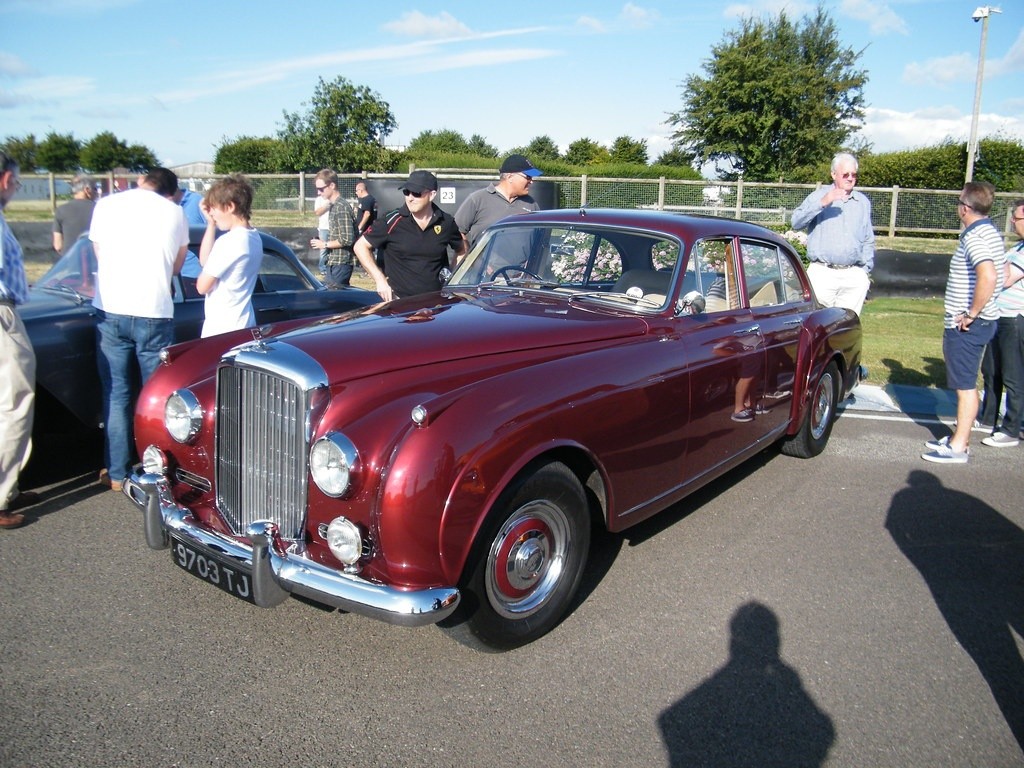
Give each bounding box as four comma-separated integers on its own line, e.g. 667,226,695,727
0,511,28,529
8,492,39,511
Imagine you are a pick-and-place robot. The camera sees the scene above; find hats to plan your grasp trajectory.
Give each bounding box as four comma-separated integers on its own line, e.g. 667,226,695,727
499,155,544,177
398,170,437,192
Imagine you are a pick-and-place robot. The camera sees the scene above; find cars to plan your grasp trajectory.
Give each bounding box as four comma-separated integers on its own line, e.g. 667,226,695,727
120,206,863,652
15,225,382,495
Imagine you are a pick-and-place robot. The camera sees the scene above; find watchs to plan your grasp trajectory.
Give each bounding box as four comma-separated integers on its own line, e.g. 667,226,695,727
964,310,975,321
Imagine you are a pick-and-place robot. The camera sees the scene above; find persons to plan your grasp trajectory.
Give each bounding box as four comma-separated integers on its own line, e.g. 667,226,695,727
88,168,190,492
954,202,1024,447
52,174,95,254
921,182,1006,465
310,168,359,286
353,170,465,303
791,154,874,318
352,182,378,239
452,154,543,279
196,178,263,339
0,149,38,529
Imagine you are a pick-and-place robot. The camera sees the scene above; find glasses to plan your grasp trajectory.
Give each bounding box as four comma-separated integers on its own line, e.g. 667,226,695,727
516,173,532,180
403,189,432,197
843,173,857,178
3,168,24,191
316,182,335,192
956,198,973,209
1011,216,1024,222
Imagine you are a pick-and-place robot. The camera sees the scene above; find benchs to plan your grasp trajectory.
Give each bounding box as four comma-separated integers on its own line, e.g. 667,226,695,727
611,268,800,308
252,274,308,294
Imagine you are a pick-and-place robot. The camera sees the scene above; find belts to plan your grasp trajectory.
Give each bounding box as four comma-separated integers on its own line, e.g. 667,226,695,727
0,298,15,306
811,261,855,269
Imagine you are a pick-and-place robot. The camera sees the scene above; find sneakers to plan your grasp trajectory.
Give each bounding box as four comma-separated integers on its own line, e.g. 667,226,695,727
100,468,123,492
731,410,753,422
921,444,968,463
981,431,1019,448
745,403,772,414
762,390,791,399
925,436,969,454
954,418,993,433
